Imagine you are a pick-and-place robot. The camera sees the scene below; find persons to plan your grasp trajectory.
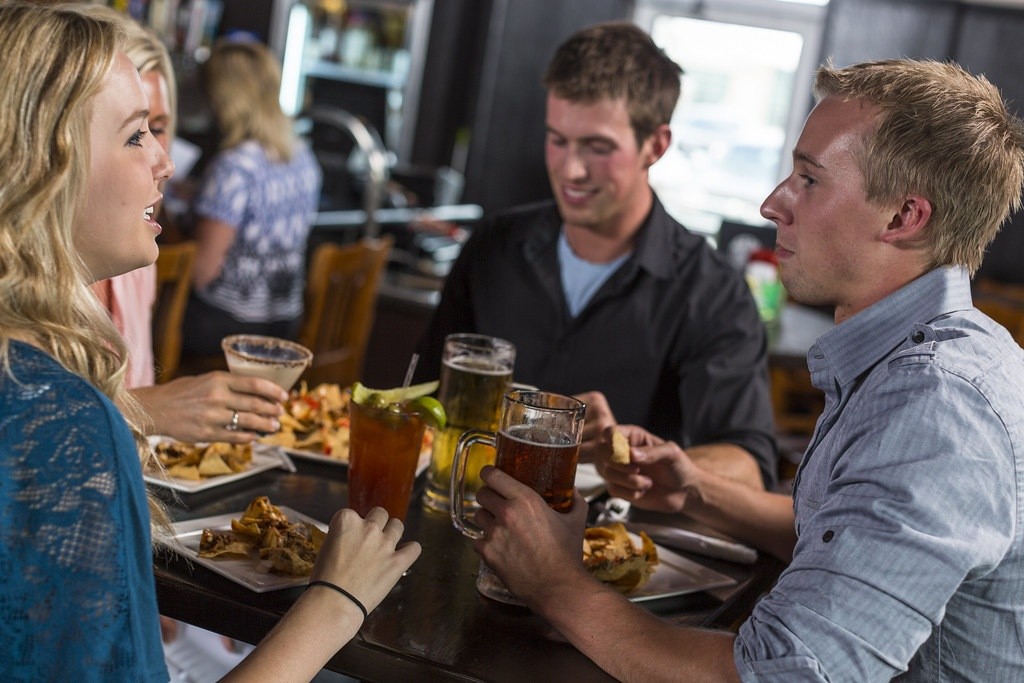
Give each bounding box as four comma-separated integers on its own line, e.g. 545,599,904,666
428,21,782,510
177,30,323,374
470,56,1024,682
12,1,250,683
0,1,422,683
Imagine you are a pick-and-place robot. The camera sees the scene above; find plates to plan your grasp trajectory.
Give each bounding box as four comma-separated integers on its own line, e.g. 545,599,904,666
136,435,282,493
574,464,641,503
279,446,433,478
151,505,330,592
626,531,737,603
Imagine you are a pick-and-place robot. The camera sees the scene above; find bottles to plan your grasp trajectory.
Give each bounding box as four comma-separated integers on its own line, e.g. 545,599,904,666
747,250,780,325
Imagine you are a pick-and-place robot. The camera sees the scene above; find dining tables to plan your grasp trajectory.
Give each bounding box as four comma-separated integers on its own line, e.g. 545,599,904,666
144,461,794,683
360,264,841,450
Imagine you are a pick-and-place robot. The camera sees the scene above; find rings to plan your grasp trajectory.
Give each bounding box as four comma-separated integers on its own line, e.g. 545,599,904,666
224,412,240,430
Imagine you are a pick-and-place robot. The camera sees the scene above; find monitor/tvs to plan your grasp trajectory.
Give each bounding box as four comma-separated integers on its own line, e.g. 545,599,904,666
295,65,402,164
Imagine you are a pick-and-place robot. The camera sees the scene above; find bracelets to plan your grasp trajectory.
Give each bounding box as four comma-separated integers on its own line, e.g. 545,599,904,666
306,581,368,624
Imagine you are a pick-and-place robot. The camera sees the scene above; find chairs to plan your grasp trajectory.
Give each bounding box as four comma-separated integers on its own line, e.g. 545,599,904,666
146,235,201,385
293,230,396,387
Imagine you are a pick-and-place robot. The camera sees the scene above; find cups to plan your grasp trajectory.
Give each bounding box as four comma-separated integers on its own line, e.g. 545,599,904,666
450,391,585,607
422,334,516,516
222,334,313,436
348,397,427,527
